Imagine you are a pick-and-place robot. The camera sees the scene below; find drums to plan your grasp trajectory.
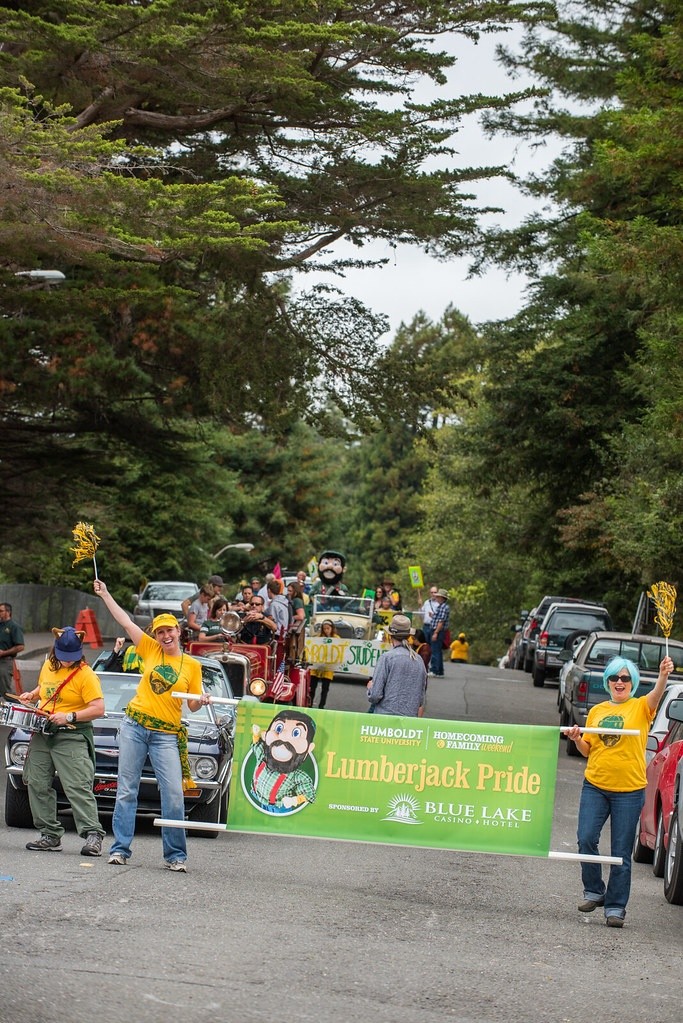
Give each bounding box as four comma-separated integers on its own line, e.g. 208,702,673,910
0,701,49,733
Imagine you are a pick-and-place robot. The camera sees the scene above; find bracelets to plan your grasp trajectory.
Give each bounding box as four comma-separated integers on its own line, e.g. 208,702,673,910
72,711,77,725
29,692,34,700
259,615,265,620
434,632,438,634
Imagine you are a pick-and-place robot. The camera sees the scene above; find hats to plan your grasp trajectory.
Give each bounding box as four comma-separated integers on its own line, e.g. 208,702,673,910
381,579,395,587
151,614,179,633
52,626,86,662
383,615,416,636
208,575,226,587
433,589,451,600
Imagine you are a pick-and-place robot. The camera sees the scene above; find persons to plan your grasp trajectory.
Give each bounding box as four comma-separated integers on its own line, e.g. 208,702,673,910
309,550,349,616
20,627,106,857
367,615,428,717
181,571,403,709
564,655,674,928
0,603,25,697
412,586,468,678
93,579,212,873
104,623,156,674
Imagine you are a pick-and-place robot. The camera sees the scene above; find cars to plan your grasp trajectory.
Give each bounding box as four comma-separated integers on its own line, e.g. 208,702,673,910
185,615,314,708
4,670,236,841
555,630,683,757
304,592,426,644
496,595,604,670
632,684,683,907
133,580,200,629
90,650,237,745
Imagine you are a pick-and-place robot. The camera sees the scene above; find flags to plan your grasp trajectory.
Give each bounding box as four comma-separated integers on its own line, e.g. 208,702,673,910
271,654,285,701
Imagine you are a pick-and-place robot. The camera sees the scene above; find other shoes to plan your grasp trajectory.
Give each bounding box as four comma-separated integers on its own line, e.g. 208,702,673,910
606,916,624,927
578,899,604,912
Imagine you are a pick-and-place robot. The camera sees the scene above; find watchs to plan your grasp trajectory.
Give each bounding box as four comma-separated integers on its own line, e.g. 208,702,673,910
66,713,73,723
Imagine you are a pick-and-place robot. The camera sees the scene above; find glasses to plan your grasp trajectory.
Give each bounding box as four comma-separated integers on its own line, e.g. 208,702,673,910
608,675,631,682
430,592,437,594
249,603,263,606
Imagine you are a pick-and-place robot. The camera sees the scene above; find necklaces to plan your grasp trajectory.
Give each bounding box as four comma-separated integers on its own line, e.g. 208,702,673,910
162,648,184,685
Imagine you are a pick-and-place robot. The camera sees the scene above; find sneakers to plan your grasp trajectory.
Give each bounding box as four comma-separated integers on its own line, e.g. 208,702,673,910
80,834,103,856
25,836,63,851
164,859,187,872
107,852,126,864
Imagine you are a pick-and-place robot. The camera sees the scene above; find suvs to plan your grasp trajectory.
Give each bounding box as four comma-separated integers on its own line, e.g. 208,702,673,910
532,602,616,687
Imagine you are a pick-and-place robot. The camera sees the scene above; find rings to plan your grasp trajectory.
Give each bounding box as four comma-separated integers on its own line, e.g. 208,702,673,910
202,703,205,705
